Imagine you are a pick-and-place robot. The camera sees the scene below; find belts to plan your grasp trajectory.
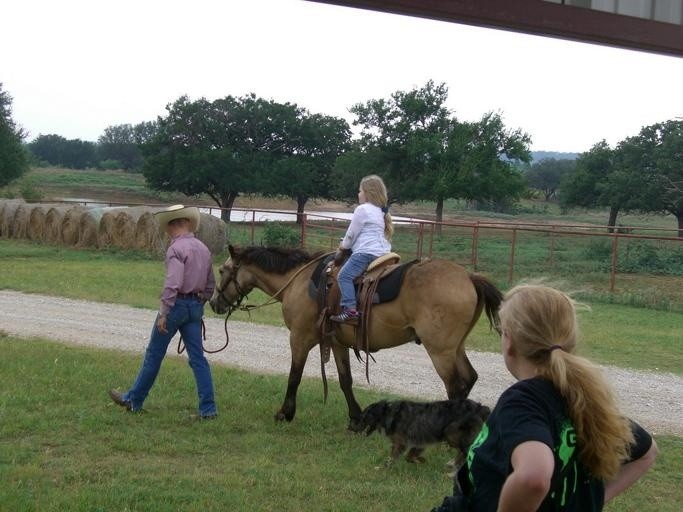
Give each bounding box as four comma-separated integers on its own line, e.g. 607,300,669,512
177,292,198,300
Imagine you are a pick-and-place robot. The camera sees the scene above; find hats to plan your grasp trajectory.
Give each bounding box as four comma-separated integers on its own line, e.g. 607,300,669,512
152,204,201,233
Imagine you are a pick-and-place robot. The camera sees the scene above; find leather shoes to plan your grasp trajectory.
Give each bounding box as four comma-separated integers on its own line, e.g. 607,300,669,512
188,414,217,420
109,388,133,412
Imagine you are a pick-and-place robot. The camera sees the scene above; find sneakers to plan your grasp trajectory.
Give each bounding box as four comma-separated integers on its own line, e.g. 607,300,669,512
329,309,359,326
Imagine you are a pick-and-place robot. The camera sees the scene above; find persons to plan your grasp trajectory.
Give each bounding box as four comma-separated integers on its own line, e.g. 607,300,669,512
109,204,217,422
430,284,659,512
329,175,393,326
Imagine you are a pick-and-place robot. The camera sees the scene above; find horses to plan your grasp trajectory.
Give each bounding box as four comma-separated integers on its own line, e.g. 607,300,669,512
206,238,504,424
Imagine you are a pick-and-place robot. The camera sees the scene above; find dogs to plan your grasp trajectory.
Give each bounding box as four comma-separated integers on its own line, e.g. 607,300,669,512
352,396,492,479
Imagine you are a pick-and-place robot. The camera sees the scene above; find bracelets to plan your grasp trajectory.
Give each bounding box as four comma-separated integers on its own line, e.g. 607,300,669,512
159,312,167,318
